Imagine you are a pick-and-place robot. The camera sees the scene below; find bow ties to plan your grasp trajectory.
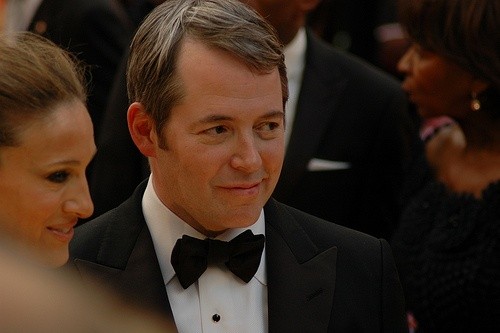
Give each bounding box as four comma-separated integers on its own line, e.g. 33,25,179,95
171,229,264,289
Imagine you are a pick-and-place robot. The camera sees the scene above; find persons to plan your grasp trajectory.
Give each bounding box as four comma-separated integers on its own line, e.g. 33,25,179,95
0,0,432,333
399,0,500,333
